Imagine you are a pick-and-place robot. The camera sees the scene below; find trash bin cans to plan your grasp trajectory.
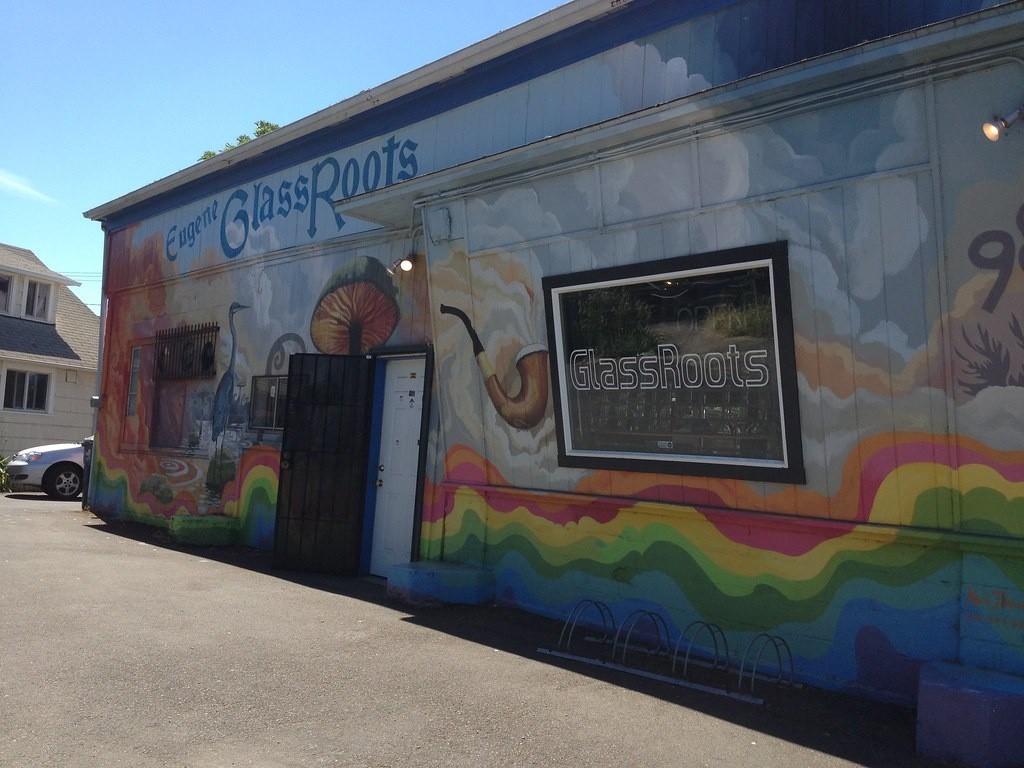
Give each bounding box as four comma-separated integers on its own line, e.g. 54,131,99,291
80,435,94,509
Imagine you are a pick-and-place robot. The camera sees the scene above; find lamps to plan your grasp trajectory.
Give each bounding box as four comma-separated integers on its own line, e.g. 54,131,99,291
982,109,1019,143
400,254,416,272
387,259,401,275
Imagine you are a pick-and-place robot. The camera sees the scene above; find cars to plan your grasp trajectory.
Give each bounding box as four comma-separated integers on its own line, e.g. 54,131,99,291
5,435,95,501
